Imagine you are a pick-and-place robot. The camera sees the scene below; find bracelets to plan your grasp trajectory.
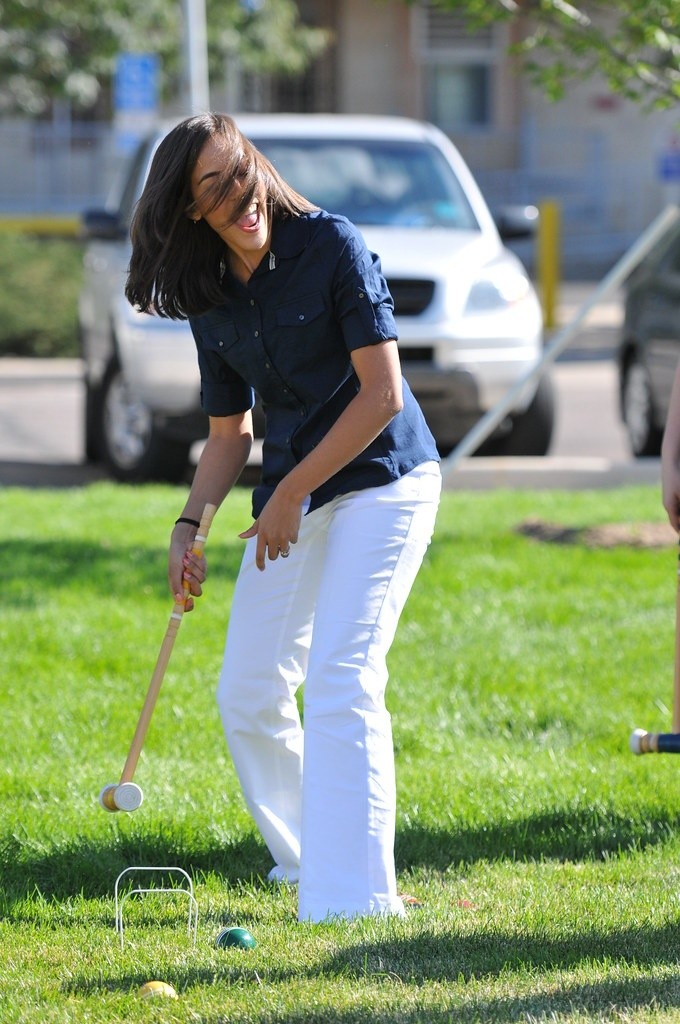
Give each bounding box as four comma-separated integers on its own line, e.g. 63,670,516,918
173,517,202,529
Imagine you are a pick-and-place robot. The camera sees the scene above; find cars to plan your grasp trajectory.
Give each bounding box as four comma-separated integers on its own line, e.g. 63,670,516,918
613,210,680,461
74,111,555,486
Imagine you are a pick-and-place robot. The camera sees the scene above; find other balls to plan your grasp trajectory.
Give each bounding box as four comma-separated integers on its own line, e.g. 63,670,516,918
216,926,255,952
139,979,176,998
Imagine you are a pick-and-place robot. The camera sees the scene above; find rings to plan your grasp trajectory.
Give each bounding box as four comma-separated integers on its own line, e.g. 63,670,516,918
280,547,290,557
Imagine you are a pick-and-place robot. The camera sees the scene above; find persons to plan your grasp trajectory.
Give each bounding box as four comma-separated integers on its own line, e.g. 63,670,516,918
125,112,446,928
